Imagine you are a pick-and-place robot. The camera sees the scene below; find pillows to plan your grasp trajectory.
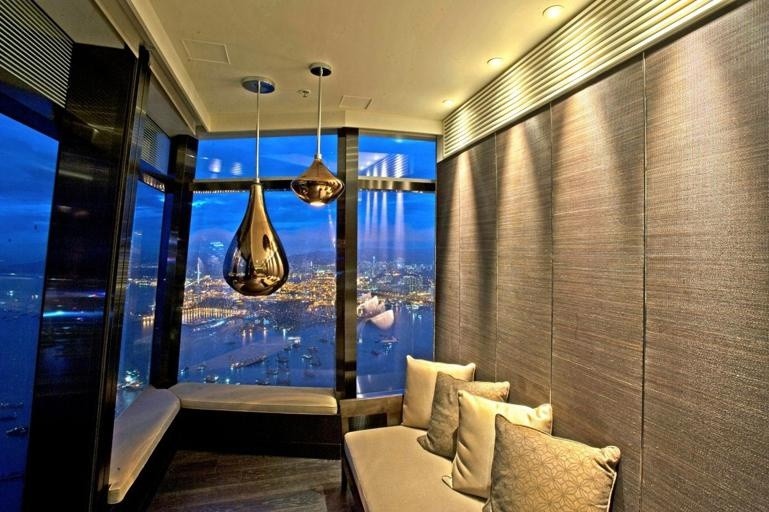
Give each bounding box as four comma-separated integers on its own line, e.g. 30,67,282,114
417,372,513,459
482,413,620,512
442,390,555,500
401,355,476,429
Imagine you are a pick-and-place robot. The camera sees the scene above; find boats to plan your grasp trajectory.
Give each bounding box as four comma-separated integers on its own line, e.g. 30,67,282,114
0,399,29,482
371,333,399,357
117,345,324,391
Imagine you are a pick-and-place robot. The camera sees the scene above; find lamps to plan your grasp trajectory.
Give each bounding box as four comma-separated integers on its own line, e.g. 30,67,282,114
222,77,289,296
290,65,345,207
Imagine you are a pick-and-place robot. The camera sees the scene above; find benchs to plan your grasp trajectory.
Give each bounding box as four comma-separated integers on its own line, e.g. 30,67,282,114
168,383,338,424
105,383,181,504
339,394,491,512
